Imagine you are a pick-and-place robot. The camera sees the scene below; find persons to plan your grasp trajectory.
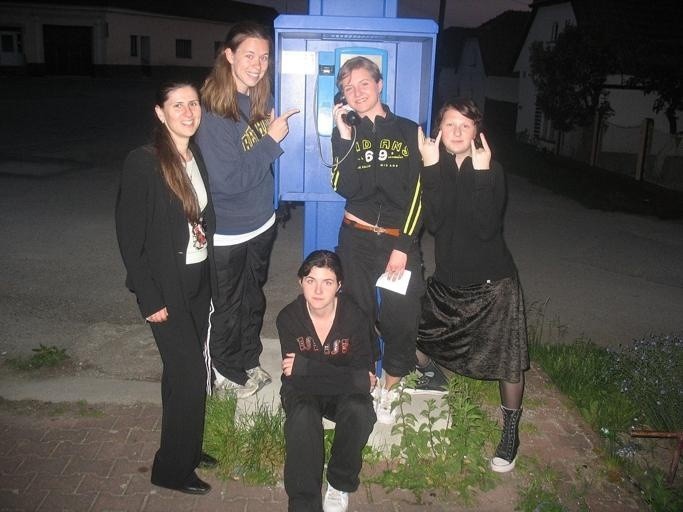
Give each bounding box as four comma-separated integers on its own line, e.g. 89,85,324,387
326,53,424,394
111,74,220,496
415,94,530,480
189,19,303,400
274,246,380,512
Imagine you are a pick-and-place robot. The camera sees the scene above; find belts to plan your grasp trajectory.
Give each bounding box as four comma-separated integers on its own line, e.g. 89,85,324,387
341,217,402,238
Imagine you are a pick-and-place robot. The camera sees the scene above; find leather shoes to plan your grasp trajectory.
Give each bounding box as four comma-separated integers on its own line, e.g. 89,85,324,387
195,452,217,469
150,467,212,495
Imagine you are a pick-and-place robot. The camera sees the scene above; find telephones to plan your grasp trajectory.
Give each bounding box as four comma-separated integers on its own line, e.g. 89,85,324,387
334,93,362,126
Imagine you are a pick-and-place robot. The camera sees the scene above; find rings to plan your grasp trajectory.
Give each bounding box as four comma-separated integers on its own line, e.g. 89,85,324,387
430,139,435,144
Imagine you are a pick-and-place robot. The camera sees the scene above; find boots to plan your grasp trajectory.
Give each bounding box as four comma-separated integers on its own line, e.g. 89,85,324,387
398,359,453,395
491,404,521,473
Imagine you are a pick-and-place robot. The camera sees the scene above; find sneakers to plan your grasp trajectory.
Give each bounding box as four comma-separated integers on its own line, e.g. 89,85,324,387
247,365,272,388
376,385,402,425
215,373,263,402
323,480,349,512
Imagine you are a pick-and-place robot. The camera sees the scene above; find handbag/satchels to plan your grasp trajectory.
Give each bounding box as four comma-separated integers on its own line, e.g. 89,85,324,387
272,194,298,227
